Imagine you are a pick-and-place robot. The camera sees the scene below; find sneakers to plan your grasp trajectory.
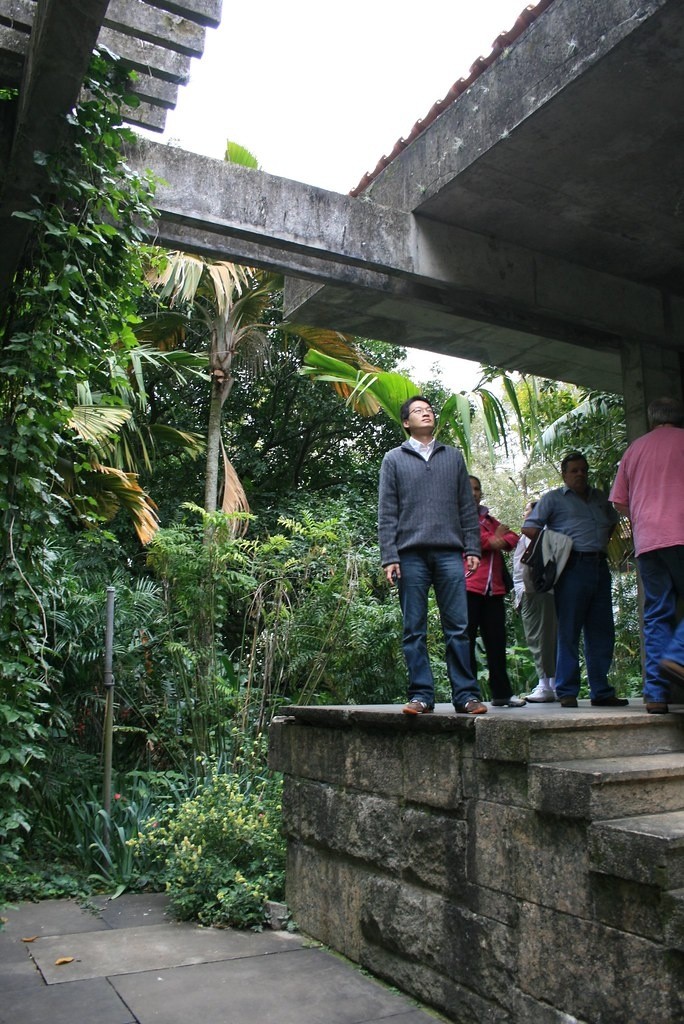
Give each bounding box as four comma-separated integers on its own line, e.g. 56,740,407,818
491,695,526,707
403,699,434,714
454,699,488,713
524,685,555,703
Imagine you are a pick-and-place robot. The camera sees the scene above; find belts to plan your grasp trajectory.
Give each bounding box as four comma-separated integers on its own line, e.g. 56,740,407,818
572,551,608,557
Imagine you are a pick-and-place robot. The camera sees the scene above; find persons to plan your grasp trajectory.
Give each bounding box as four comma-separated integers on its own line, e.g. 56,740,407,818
377,396,487,715
513,502,559,703
521,453,629,707
462,475,526,707
607,398,684,714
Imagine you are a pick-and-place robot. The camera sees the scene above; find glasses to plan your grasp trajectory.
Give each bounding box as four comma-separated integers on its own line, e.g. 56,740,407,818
408,408,433,414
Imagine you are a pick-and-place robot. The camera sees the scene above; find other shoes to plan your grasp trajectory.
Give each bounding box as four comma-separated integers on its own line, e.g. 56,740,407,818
655,659,684,688
591,698,628,706
646,702,668,713
561,695,577,707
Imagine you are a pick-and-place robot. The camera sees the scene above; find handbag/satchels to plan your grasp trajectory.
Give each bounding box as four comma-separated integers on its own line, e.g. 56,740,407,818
502,561,514,590
